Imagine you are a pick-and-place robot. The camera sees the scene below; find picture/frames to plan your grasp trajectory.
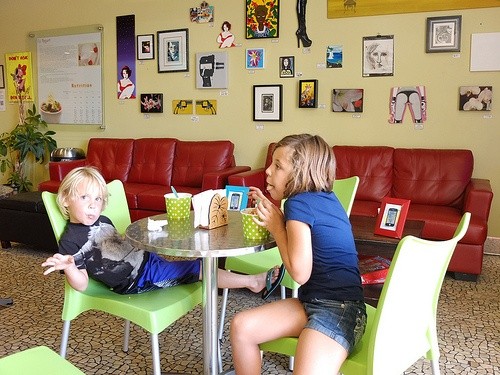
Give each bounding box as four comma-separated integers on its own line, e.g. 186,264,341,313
157,28,188,73
362,35,394,77
425,15,462,53
253,85,282,122
299,79,318,109
137,34,154,60
246,48,264,69
279,56,295,78
225,185,249,211
375,198,412,240
246,0,281,40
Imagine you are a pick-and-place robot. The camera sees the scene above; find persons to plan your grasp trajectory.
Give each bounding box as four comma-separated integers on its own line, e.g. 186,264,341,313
41,166,286,300
231,133,367,375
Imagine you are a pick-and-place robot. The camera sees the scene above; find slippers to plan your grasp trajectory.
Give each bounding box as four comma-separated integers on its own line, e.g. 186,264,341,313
261,263,286,300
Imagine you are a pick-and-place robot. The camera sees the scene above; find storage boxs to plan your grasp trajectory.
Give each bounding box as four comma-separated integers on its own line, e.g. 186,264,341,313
193,189,229,230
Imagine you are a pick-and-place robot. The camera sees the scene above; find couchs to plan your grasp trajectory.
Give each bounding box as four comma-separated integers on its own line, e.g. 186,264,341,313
228,143,494,282
39,137,252,224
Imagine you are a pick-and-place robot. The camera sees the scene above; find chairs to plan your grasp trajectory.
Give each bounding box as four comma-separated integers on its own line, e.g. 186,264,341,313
258,212,471,375
217,176,359,373
41,179,204,375
0,345,85,375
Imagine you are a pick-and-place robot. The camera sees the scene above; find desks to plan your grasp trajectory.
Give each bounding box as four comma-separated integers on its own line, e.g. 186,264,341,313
348,215,424,301
125,210,276,375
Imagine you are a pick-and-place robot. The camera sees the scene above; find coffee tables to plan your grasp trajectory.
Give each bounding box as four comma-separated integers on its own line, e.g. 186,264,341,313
0,192,58,254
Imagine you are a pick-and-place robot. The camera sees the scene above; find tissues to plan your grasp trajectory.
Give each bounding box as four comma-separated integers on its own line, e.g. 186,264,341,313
191,189,229,230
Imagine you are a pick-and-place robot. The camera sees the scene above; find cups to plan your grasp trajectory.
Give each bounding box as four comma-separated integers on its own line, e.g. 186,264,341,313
40,105,63,124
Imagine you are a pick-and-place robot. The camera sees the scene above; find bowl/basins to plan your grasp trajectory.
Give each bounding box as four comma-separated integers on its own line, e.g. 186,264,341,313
167,221,192,240
240,207,270,239
164,192,192,219
